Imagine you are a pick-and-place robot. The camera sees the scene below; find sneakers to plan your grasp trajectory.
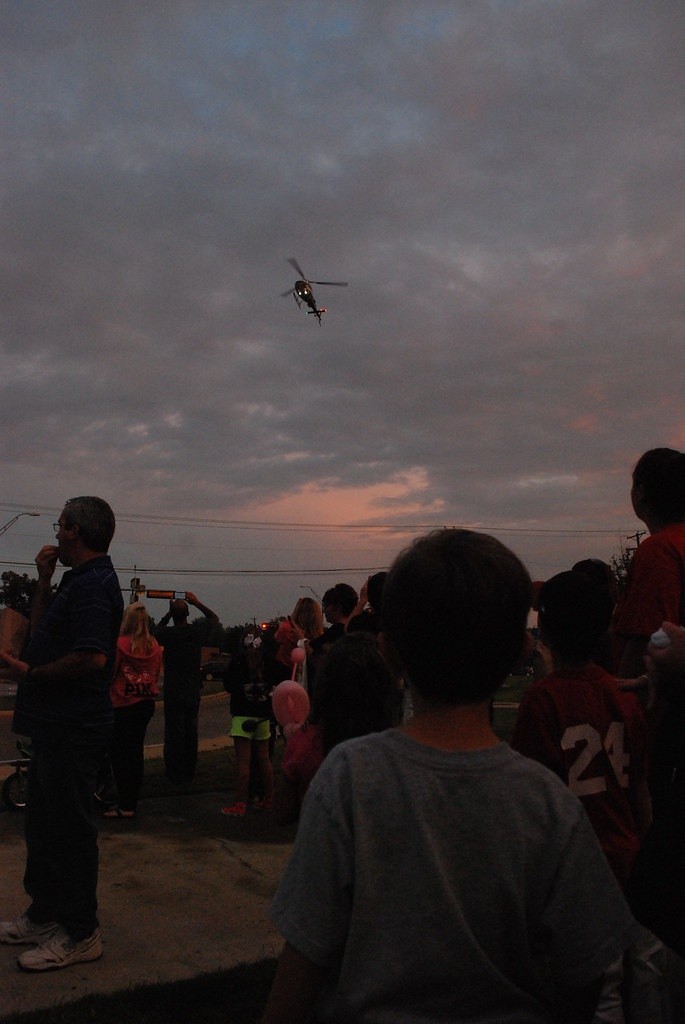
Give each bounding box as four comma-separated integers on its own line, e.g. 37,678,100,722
1,919,49,943
221,803,246,816
257,801,272,809
17,927,104,971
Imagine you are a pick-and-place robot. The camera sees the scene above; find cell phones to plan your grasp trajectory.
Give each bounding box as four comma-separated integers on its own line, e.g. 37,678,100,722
172,592,187,600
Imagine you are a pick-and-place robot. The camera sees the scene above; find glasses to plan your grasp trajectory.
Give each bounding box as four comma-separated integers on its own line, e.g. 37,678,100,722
53,522,75,531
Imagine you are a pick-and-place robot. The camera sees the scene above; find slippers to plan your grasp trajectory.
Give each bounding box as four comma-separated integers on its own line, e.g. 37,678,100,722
103,808,135,819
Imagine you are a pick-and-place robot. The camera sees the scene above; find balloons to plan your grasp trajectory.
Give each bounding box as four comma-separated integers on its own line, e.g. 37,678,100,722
274,678,310,733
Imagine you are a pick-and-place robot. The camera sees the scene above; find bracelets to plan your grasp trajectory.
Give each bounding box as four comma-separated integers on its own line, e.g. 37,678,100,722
24,665,33,679
640,674,649,682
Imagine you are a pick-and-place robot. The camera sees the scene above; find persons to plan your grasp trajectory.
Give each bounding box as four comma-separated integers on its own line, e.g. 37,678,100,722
105,601,161,819
154,593,218,781
0,496,124,973
225,447,685,1024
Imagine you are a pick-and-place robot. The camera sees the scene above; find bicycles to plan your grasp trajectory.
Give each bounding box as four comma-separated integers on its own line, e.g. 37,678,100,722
2,739,125,811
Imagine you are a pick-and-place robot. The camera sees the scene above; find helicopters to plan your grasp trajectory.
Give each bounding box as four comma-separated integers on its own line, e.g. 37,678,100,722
279,255,350,328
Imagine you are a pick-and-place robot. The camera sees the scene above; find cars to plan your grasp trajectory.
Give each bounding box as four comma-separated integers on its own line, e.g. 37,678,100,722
198,658,240,682
